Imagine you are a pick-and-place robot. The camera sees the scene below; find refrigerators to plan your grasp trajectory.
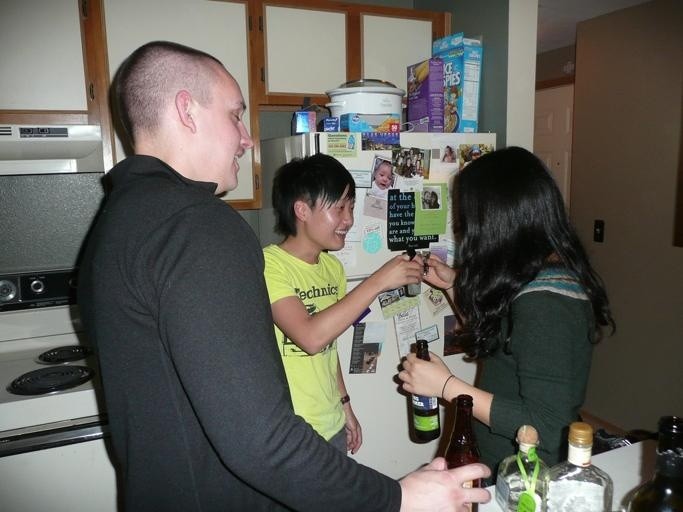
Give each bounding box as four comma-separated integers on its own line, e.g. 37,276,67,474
259,131,498,479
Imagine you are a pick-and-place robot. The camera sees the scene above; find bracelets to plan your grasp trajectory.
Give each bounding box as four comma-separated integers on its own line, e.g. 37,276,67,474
439,373,454,401
444,284,454,292
339,393,350,405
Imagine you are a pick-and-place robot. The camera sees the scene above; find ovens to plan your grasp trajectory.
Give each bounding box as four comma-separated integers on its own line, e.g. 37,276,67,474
0,422,120,512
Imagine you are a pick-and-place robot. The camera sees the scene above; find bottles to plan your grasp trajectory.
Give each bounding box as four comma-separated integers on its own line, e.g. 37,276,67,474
626,415,682,512
542,422,613,512
407,339,441,444
494,426,549,511
443,395,482,512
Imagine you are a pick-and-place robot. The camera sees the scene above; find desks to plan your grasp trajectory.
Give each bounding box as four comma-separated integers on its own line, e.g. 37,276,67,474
480,432,659,512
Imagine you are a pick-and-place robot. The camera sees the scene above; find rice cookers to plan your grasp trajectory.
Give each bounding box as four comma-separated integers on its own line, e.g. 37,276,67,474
323,78,407,131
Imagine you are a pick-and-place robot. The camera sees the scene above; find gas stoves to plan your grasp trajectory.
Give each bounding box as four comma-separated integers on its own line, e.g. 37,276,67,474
0,332,113,436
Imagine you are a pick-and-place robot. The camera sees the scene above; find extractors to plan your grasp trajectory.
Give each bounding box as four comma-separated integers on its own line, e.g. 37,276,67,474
0,123,106,178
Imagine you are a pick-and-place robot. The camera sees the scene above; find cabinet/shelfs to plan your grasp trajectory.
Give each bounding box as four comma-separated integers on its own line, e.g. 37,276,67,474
0,2,100,125
82,1,264,211
248,0,454,112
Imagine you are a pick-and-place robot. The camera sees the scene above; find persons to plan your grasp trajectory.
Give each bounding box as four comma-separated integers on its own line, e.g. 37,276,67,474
259,152,425,460
368,143,491,209
395,144,617,487
71,40,494,512
